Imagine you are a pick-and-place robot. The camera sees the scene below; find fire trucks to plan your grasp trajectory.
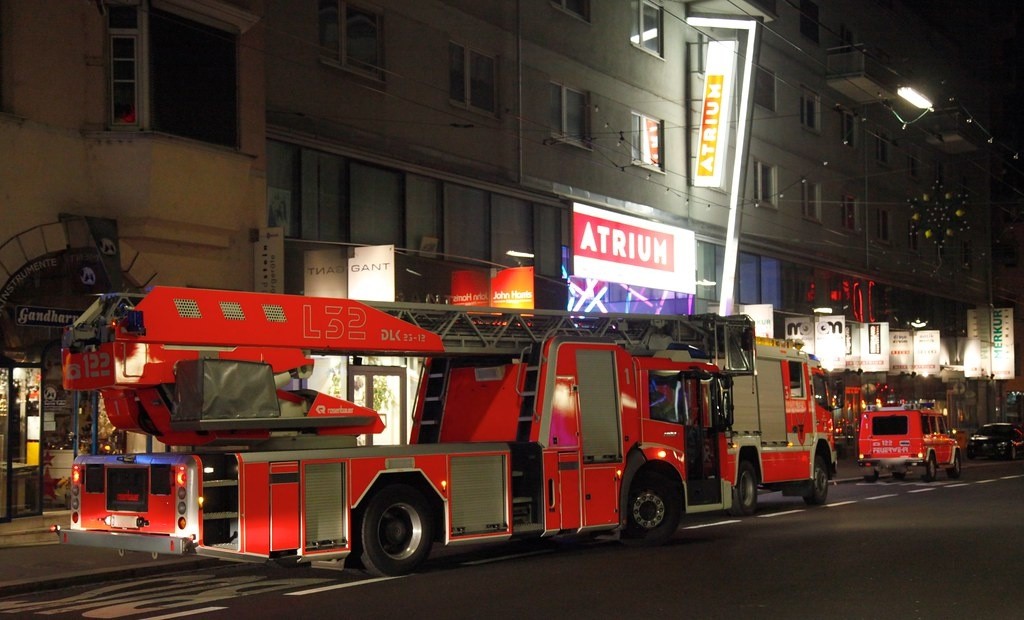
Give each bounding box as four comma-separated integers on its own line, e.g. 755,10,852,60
60,286,846,580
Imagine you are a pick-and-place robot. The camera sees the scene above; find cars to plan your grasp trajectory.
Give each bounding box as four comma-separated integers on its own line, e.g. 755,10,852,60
967,422,1024,461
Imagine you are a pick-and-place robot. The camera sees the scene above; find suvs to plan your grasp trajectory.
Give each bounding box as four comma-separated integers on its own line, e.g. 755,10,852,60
858,402,961,483
